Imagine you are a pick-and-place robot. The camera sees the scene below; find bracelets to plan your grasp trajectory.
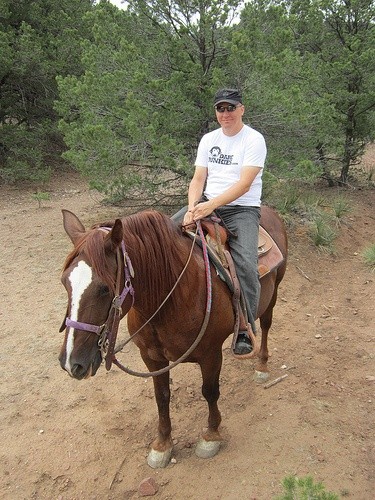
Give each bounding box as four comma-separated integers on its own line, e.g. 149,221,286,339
188,209,194,213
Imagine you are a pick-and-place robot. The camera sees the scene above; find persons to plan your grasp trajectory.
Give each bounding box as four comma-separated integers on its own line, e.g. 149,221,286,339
165,86,268,356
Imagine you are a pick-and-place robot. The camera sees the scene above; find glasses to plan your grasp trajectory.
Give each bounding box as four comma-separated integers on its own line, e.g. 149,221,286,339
215,105,238,112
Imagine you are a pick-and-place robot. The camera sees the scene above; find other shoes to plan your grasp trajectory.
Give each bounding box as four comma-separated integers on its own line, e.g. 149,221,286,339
235,330,256,354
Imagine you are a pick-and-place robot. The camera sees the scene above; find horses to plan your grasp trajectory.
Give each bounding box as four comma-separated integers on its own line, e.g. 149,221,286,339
58,204,288,469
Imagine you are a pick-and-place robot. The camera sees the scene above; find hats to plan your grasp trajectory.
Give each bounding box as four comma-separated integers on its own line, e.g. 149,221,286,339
214,87,243,108
